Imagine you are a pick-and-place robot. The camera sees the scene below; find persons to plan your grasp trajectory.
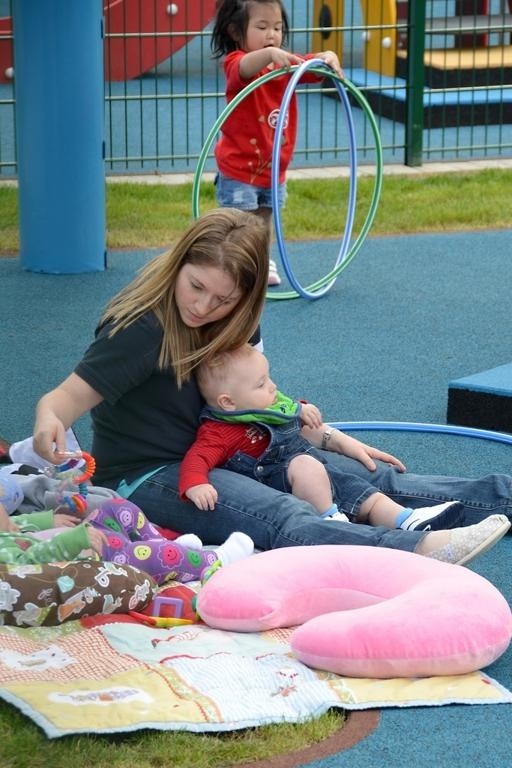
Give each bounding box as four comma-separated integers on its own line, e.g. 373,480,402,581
211,1,346,290
177,344,466,536
1,493,255,587
32,204,512,574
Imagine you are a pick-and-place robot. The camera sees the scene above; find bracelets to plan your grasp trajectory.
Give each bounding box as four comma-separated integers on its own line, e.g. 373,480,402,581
321,426,337,450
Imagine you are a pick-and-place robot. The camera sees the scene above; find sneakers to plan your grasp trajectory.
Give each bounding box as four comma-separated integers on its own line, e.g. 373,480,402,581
401,500,464,530
268,259,280,286
426,514,511,565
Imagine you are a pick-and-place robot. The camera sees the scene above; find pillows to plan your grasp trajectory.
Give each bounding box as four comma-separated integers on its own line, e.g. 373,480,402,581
1,558,157,627
192,545,512,678
